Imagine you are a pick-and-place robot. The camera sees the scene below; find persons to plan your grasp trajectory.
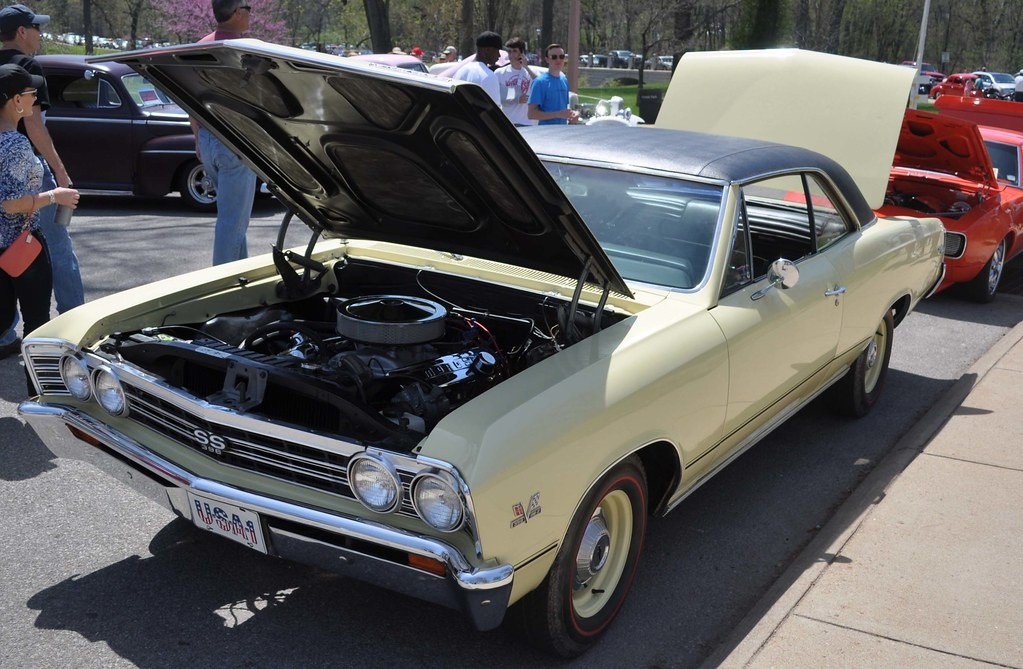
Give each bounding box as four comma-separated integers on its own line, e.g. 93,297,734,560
973,75,983,90
188,0,257,267
318,30,580,126
0,4,84,315
0,63,79,400
964,80,971,97
1015,69,1023,102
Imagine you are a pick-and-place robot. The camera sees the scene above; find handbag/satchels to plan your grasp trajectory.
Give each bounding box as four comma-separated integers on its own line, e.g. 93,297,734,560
0,228,42,279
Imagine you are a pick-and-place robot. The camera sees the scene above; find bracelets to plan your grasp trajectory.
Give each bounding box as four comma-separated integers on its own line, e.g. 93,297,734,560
48,190,55,205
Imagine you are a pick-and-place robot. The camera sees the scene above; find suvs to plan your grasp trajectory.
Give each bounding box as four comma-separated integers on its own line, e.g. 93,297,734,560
900,60,945,86
609,50,641,68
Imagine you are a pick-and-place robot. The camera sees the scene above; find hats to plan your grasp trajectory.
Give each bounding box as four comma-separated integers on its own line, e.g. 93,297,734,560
0,4,50,42
392,47,407,54
0,64,43,111
411,47,424,55
1019,69,1023,74
442,46,458,53
475,31,503,49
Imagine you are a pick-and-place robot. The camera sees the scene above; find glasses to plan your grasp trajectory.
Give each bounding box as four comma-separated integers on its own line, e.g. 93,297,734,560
21,88,38,96
551,54,566,60
234,6,251,13
29,22,41,31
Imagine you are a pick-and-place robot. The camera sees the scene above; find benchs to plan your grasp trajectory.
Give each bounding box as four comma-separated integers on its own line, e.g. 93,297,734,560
50,99,97,109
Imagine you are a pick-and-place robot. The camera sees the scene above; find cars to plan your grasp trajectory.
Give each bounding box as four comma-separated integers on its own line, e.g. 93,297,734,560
42,33,169,51
877,95,1023,305
644,56,674,70
930,73,992,100
23,38,946,663
25,54,217,212
971,72,1016,98
300,45,371,57
578,54,608,67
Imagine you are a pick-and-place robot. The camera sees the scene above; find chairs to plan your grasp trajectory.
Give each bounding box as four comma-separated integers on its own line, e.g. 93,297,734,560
580,186,818,299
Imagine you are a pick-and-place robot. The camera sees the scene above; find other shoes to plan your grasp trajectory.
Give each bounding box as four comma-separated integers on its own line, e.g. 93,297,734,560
0,310,20,346
